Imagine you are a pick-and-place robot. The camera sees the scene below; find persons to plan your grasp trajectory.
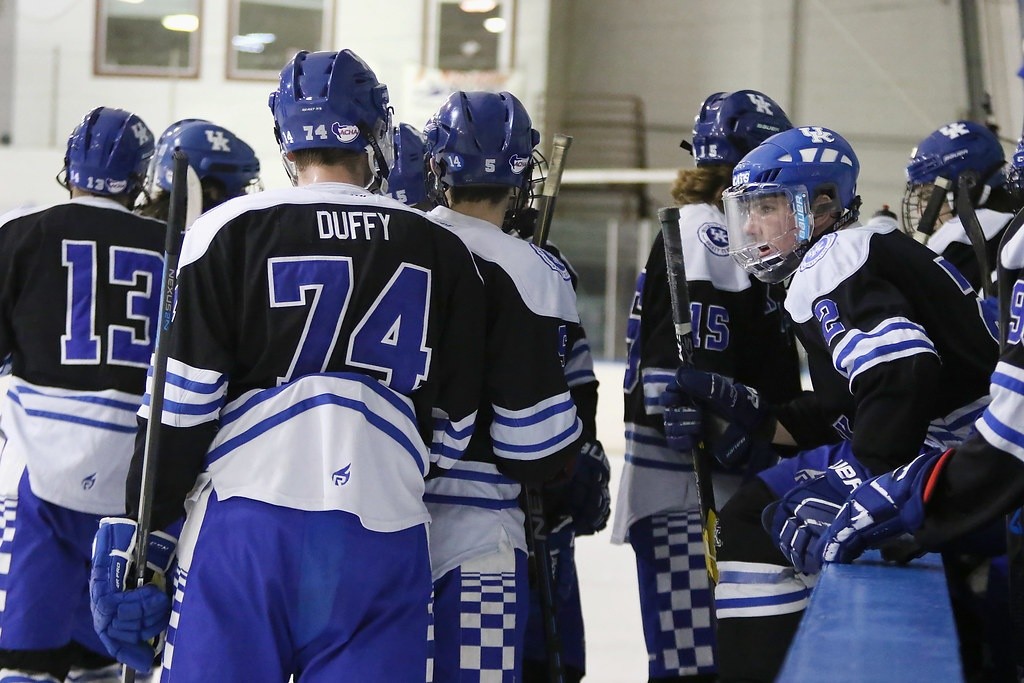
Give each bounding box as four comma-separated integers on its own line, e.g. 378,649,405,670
90,49,485,683
134,119,262,229
609,89,793,683
378,89,610,683
661,121,1024,683
0,105,182,683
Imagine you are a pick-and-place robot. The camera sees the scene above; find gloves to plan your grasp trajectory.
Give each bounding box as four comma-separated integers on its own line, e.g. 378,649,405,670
89,516,172,672
661,364,764,450
821,451,944,566
767,456,870,575
559,440,612,534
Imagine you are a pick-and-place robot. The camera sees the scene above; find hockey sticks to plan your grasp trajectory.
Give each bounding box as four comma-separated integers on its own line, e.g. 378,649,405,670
661,206,717,630
121,151,188,683
922,170,1024,354
521,476,565,683
534,135,574,242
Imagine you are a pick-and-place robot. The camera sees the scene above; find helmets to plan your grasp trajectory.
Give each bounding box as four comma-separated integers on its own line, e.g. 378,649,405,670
147,119,263,210
56,107,154,199
691,90,795,168
722,125,860,283
268,50,394,157
901,120,1006,244
424,90,535,219
503,149,552,233
375,120,449,213
